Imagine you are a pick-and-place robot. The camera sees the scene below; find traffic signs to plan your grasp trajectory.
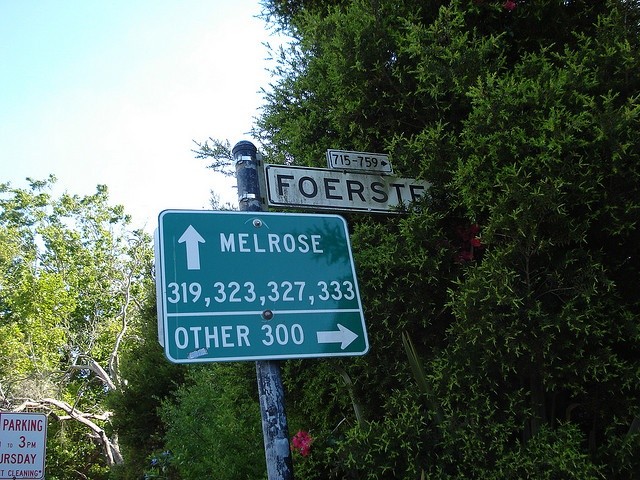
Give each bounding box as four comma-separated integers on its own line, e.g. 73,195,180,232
154,208,370,364
263,151,437,216
